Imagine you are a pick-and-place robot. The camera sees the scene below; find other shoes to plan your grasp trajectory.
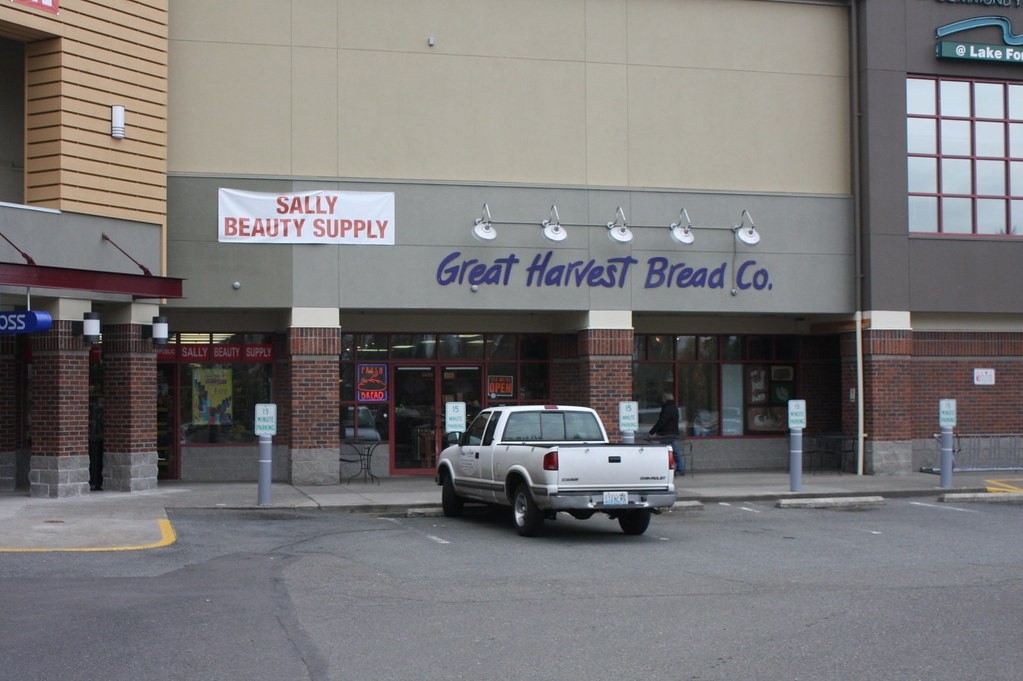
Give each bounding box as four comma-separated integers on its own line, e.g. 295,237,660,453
674,470,686,476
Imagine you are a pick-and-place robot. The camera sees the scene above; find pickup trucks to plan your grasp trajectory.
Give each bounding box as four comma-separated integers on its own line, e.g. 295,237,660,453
344,405,382,441
435,403,680,534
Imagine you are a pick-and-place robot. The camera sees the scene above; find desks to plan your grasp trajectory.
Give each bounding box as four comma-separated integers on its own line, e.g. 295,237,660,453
344,439,383,486
643,435,693,475
807,435,857,476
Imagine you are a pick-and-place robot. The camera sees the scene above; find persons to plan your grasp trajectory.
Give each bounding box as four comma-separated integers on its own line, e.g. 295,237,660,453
649,391,686,476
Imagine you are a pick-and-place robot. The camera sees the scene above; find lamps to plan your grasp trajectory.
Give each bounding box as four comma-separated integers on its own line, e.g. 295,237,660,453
111,104,125,139
670,208,695,246
73,312,100,342
471,202,498,242
732,210,761,246
541,204,568,243
607,206,634,245
142,316,168,345
